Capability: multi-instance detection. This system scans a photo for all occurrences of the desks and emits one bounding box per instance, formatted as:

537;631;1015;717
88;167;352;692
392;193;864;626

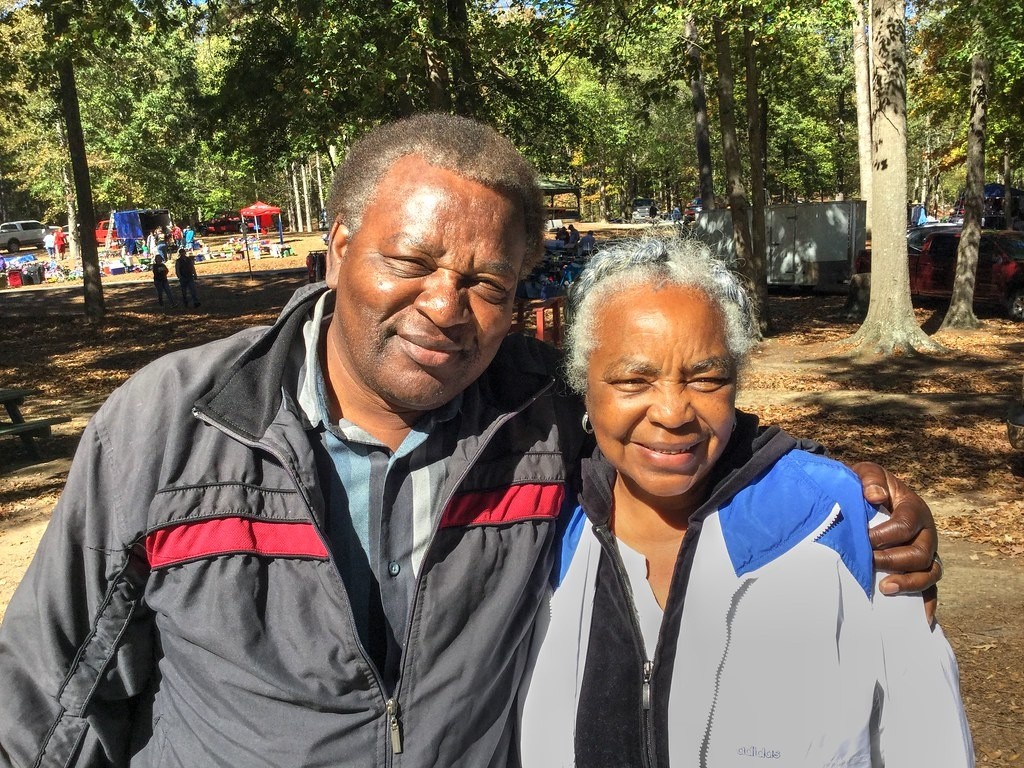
0;387;46;461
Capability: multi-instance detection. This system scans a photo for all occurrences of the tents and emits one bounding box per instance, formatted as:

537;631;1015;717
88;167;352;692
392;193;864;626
241;201;283;244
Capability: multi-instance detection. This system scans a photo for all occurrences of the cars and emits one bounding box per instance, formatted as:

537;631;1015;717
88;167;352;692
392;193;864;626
627;198;659;224
48;226;61;233
683;197;703;221
62;224;82;246
906;223;965;254
196;210;254;236
95;220;118;247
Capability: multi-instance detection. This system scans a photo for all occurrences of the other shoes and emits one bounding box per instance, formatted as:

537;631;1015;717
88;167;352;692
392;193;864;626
159;301;163;306
170;303;178;308
194;301;202;308
183;302;189;308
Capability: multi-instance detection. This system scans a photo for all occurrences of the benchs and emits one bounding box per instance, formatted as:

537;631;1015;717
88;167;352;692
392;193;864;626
0;416;72;447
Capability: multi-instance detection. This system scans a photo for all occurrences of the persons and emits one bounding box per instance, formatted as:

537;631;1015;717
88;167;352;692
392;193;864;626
625;203;634;223
153;255;177;308
72;224;81;258
671;204;680;227
175;249;201;307
513;235;975;768
54;227;68;260
649;202;659;217
0;112;941;768
142;223;194;263
555;225;595;255
42;229;56;259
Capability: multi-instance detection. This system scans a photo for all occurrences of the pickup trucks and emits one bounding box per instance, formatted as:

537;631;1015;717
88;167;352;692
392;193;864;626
856;228;1024;322
1;220;50;253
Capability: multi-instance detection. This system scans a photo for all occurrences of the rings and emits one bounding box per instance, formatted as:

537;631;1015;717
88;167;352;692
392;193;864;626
935;559;945;576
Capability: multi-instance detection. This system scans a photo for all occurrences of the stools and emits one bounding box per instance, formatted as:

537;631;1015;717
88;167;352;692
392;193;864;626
506;295;564;346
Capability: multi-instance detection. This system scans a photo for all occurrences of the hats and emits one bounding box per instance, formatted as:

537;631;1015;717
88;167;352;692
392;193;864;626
548;276;553;281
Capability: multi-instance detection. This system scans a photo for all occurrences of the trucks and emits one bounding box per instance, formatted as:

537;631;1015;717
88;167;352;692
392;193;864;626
681;199;867;286
